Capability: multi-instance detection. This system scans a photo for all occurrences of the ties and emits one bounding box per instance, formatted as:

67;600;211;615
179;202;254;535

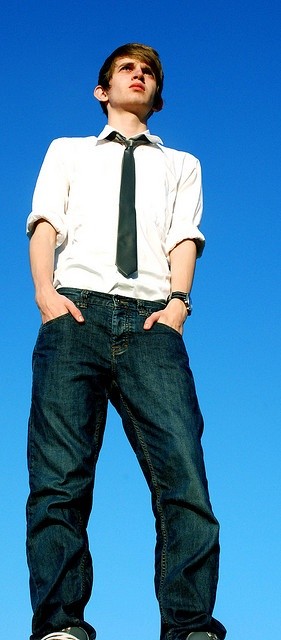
104;130;153;277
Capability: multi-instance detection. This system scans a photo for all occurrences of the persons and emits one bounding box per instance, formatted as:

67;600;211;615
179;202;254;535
25;43;228;640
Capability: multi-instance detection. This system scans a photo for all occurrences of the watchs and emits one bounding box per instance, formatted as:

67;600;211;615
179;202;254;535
167;291;192;316
